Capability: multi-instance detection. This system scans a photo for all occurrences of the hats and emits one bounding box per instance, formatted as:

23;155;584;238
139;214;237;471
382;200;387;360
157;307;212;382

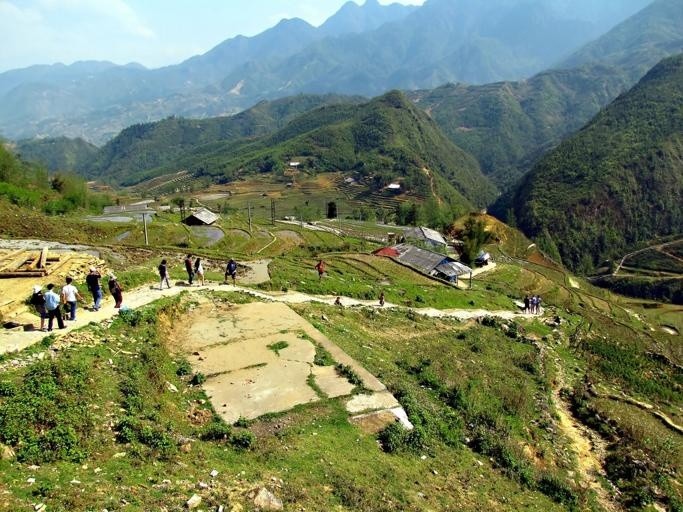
110;275;117;281
90;267;96;272
33;285;42;294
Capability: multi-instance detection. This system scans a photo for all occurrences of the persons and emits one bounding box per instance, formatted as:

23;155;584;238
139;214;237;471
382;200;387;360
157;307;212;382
225;258;237;287
31;285;46;331
524;295;541;314
185;254;195;284
158;260;171;290
195;258;204;287
109;275;122;308
61;277;83;320
43;284;67;331
315;259;325;280
86;267;101;311
379;293;384;306
334;297;342;305
